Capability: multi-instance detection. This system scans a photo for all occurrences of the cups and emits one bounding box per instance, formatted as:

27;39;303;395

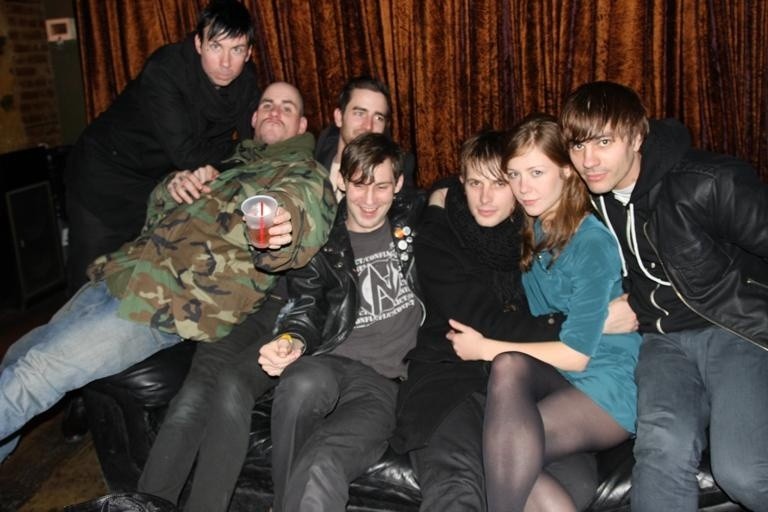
241;194;280;251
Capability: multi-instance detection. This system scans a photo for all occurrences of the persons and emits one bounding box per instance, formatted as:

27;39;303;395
386;130;640;512
138;77;392;511
562;81;768;512
259;133;448;511
0;81;336;460
63;1;264;443
445;110;642;511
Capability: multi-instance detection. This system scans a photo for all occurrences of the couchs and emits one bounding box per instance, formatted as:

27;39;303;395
80;308;753;512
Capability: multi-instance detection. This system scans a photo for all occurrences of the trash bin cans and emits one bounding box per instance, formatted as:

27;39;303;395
0;146;69;312
